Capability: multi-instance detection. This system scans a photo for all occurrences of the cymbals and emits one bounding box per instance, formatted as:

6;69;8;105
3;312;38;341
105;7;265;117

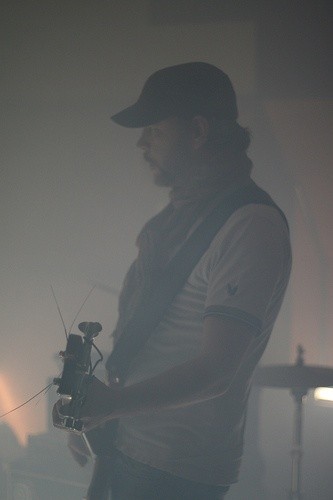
253;364;333;387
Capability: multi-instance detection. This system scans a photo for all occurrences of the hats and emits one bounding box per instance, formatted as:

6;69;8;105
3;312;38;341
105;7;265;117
109;61;239;128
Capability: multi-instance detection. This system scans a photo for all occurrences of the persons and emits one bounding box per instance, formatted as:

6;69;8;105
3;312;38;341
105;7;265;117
51;62;293;500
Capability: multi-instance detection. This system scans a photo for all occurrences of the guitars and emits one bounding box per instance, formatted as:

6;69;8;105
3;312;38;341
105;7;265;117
54;321;105;433
